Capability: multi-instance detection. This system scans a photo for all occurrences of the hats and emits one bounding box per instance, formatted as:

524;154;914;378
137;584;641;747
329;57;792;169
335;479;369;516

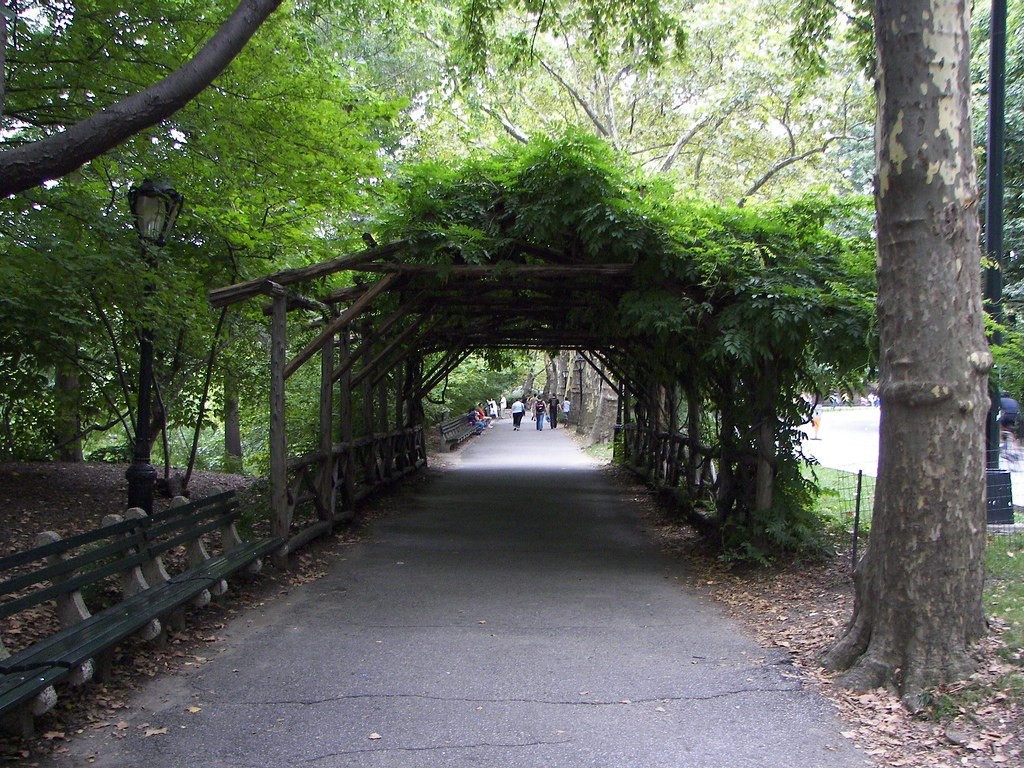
537;395;542;399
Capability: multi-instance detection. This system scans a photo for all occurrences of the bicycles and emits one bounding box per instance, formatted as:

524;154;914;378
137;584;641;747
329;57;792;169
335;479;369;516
1000;424;1023;472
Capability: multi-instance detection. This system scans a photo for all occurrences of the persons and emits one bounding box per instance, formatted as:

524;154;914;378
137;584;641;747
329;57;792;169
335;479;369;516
466;403;494;435
510;398;526;431
547;393;562;429
486;397;499;419
527;392;547;431
499;393;507;419
561;396;575;428
999;389;1021;447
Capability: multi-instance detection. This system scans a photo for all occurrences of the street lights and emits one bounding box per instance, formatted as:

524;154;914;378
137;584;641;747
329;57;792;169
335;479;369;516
117;173;186;534
561;366;568;389
575;356;587;430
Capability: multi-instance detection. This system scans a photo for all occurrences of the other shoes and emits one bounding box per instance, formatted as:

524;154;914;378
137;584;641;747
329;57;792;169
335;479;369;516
487;424;493;428
534;419;536;421
514;427;516;430
516;429;520;431
531;417;533;420
564;423;569;428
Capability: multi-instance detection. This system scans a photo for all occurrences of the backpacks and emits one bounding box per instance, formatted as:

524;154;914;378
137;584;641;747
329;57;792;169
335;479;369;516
550;398;557;407
536;400;546;412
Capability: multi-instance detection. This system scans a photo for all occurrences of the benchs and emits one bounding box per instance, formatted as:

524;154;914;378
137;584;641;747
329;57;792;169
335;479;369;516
439;413;489;453
0;489;280;739
